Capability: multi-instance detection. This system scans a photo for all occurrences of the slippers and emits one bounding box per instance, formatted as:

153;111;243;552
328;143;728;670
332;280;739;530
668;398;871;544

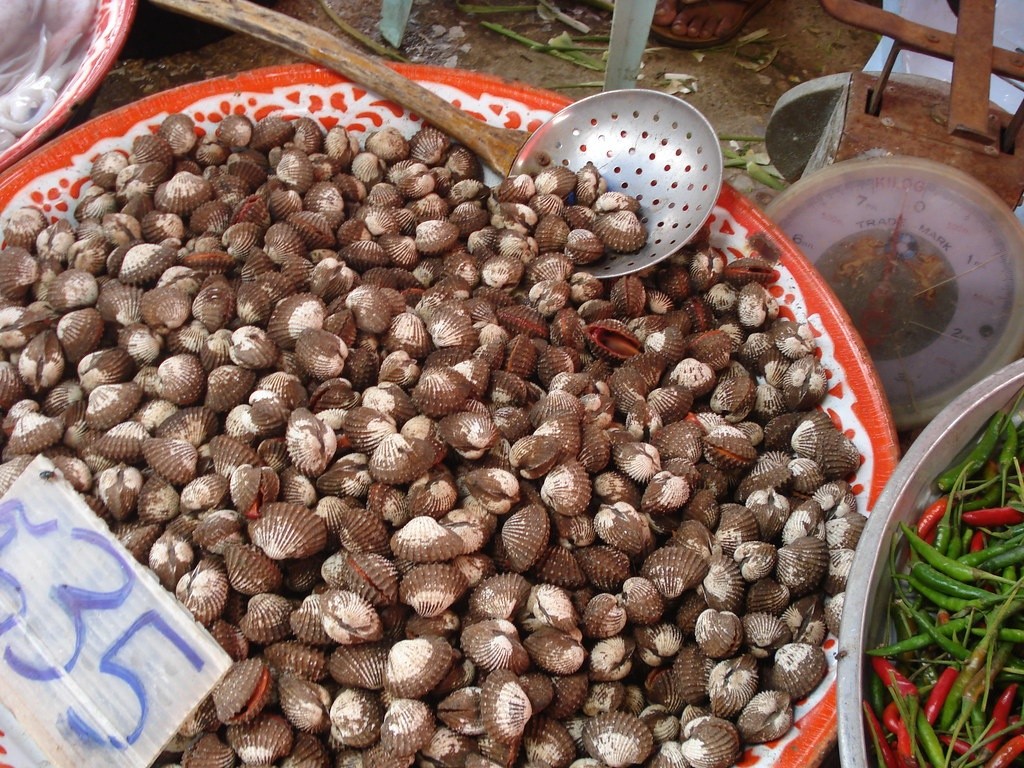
651;2;771;50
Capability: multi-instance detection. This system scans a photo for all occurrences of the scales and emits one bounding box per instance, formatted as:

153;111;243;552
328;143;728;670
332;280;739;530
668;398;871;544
759;0;1024;429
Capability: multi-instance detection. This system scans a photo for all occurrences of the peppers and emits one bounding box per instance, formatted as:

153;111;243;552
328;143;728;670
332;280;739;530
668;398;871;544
860;388;1024;768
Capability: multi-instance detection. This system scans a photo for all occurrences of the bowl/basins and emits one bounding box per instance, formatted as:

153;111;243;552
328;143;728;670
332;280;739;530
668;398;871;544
0;0;138;171
838;358;1024;768
0;63;900;768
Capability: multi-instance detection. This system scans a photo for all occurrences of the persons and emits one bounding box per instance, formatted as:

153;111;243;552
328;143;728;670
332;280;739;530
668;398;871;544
649;0;768;49
